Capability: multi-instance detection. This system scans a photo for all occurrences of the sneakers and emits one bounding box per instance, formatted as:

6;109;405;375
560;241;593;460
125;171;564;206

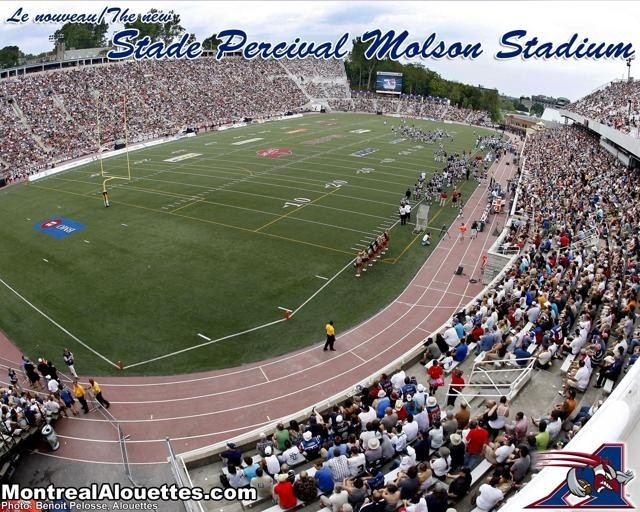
81;405;89;415
324;348;336;351
98;403;110;409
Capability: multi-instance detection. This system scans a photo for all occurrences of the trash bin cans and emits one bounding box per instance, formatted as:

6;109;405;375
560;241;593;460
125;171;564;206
41;424;60;451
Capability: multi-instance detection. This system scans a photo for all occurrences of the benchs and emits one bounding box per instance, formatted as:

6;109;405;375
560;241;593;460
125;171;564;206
1;400;61;456
223;295;630;511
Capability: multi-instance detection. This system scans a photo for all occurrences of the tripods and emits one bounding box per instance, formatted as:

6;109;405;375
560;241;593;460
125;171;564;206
439;227;450;240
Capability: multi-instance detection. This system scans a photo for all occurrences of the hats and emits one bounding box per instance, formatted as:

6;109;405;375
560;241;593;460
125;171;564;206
45;374;51;379
30;398;35;403
227;366;463;490
21;392;25;397
